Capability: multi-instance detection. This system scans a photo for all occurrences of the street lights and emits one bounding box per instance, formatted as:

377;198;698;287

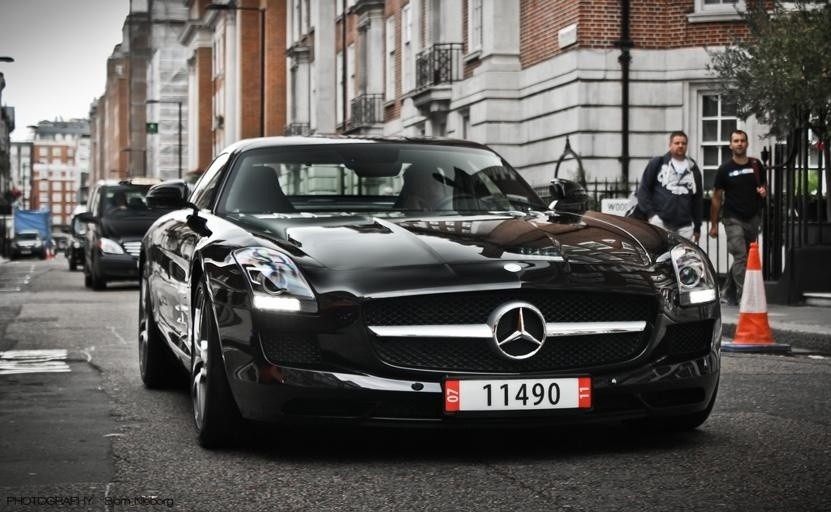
145;99;183;180
111;169;131;180
0;55;15;63
121;147;147;178
23;161;46;209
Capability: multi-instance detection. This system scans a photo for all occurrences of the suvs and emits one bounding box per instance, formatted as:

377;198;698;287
8;229;48;260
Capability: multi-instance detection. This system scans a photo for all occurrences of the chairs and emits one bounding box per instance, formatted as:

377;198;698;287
233;166;296;214
392;163;448;213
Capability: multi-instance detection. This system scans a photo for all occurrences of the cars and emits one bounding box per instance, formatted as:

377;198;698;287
138;133;724;447
80;177;190;292
48;223;73;251
61;206;87;272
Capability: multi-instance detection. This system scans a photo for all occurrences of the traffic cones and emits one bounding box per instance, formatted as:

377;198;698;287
51;248;56;260
720;242;793;354
45;248;52;261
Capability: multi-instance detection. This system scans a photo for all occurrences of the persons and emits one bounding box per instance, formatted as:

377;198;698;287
397;160;448;211
710;132;769;306
633;132;703;241
105;191;129;215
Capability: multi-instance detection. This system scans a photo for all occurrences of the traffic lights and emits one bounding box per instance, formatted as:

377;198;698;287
145;123;158;134
